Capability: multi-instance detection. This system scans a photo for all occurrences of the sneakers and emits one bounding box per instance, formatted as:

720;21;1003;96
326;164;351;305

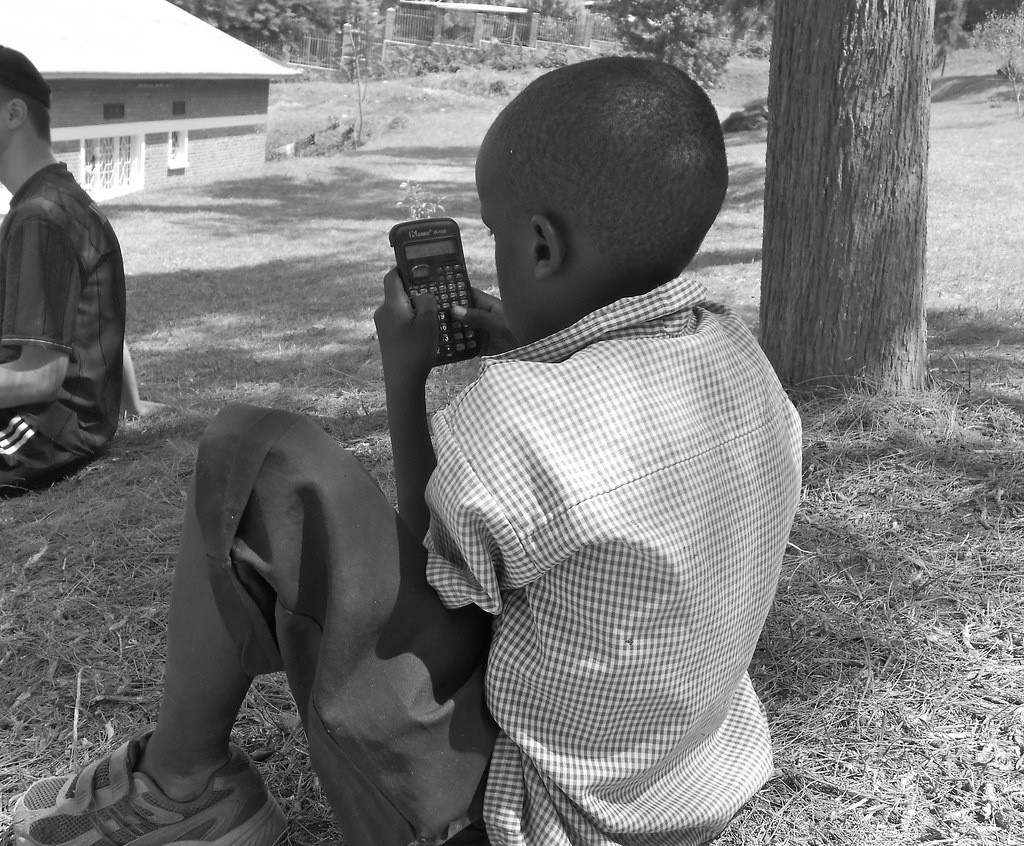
13;729;288;846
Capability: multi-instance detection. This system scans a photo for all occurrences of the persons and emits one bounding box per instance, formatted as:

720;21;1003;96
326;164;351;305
13;56;801;846
0;41;128;496
